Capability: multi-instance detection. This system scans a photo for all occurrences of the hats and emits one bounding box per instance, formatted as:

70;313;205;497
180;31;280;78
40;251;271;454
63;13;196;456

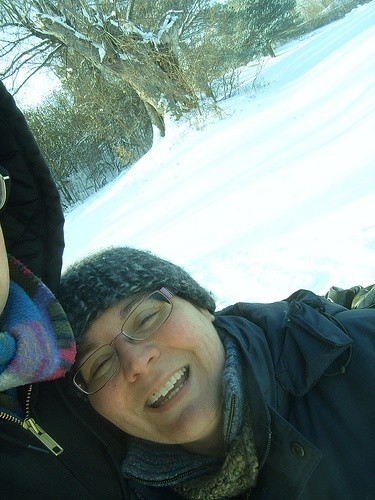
58;246;216;341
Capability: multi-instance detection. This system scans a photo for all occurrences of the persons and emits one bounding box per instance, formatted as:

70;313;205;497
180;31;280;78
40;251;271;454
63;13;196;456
0;82;375;500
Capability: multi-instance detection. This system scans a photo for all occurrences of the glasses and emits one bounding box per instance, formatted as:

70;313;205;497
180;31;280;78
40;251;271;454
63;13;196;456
0;174;9;209
72;287;174;395
